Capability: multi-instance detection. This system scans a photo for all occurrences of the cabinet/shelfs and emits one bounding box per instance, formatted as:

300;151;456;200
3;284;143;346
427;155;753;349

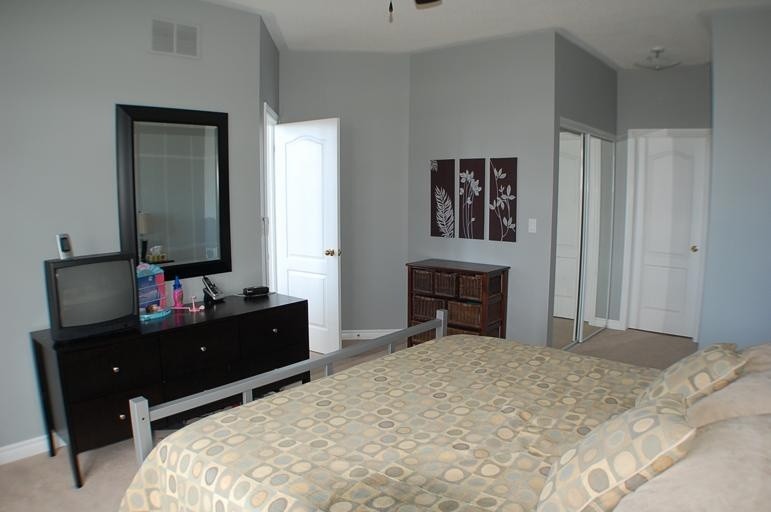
406;259;511;348
31;291;311;488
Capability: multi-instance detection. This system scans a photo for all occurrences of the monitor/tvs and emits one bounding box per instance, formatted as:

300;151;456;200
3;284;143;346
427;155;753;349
43;252;140;342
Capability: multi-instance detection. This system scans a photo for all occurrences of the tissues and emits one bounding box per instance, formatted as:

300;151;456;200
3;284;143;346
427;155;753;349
146;246;167;263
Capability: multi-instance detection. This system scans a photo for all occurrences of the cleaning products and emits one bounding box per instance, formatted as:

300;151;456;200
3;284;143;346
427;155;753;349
173;276;183;307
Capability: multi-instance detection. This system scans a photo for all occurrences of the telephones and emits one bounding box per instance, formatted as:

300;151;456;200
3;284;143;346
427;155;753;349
202;272;225;304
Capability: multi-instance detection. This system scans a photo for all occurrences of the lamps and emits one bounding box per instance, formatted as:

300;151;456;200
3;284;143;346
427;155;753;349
136;213;153;259
635;46;680;70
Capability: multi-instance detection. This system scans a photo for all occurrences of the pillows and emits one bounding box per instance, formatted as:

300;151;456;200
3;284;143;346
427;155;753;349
537;343;771;512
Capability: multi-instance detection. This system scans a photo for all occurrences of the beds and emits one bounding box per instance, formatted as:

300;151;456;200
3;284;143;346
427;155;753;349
118;308;771;512
